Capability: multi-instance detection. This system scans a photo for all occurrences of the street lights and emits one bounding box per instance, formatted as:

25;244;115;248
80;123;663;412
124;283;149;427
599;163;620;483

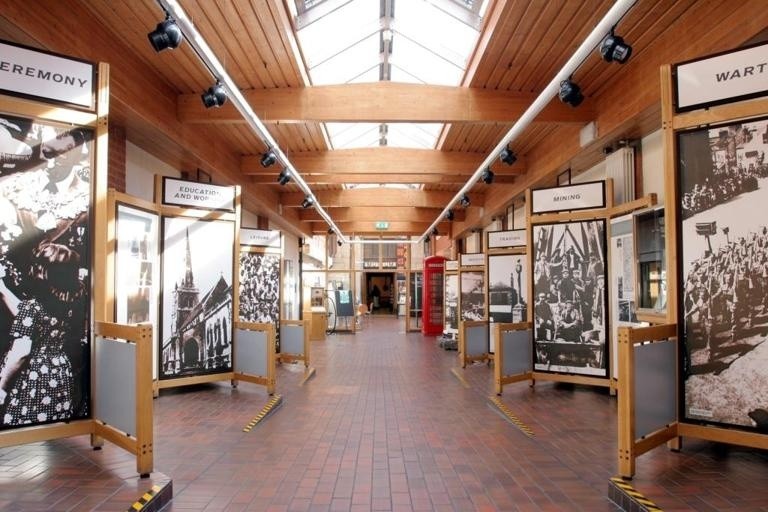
515;260;523;304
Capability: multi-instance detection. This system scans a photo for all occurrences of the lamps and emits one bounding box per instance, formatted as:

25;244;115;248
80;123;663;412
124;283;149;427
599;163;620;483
481;166;497;184
259;144;313;211
599;33;633;64
201;76;228;108
556;80;584;108
460;192;470;207
147;12;181;52
501;145;516;166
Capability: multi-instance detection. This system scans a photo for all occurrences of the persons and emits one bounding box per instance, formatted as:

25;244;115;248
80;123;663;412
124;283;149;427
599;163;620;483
0;118;90;430
239;254;280;322
680;150;768;372
534;245;603;342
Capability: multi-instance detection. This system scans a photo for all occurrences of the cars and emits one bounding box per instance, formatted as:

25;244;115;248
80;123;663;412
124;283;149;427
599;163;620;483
490;286;512;321
